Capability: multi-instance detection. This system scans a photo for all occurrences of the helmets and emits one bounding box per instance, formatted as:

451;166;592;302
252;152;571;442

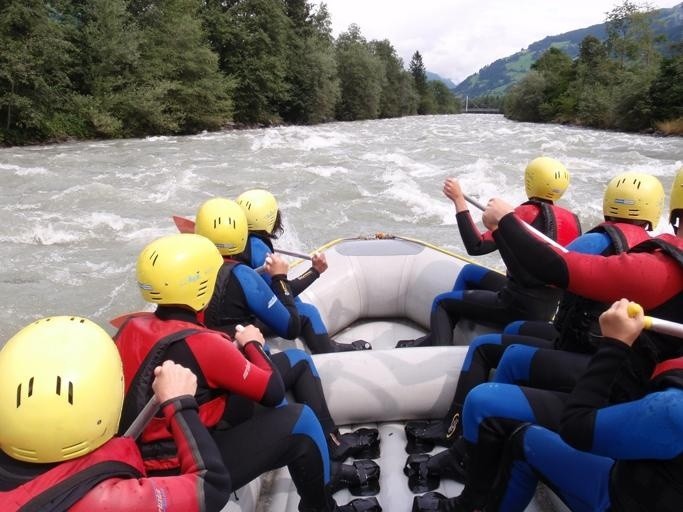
524;157;571;202
237;188;278;235
669;167;683;234
0;317;124;462
195;198;248;256
138;234;224;311
604;173;665;231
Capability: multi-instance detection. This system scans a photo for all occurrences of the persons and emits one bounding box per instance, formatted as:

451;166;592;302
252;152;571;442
396;156;581;347
114;233;379;512
1;315;231;512
195;197;378;462
410;300;683;511
233;190;372;353
400;165;683;493
405;171;665;454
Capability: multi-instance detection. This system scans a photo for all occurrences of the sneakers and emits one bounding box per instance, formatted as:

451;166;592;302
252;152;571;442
328;340;371;352
412;492;478;511
328;428;378;463
333;498;382;512
330;460;380;497
396;335;433;347
405;415;463;447
410;450;477;483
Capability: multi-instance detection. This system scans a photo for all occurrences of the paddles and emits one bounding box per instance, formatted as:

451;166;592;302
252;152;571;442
172;215;314;262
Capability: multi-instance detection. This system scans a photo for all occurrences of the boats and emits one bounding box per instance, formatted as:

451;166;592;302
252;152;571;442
210;233;562;511
